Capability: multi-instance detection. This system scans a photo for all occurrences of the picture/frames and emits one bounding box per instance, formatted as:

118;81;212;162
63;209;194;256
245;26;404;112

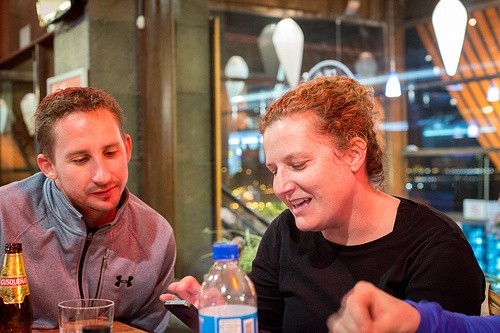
46;67;88;96
212;2;392;275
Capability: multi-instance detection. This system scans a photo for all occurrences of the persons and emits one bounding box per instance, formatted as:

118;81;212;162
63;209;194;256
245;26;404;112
0;88;177;333
159;76;486;332
326;280;500;332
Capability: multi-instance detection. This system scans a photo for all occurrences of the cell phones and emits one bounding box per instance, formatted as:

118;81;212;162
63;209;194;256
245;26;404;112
164;300;199;331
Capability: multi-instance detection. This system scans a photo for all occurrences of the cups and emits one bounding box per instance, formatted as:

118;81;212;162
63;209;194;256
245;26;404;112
58;299;114;333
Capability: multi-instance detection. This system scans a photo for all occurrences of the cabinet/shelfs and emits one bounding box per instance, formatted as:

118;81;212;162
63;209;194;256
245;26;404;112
0;0;53;187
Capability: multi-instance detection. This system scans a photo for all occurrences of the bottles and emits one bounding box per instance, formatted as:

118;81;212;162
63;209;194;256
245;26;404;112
197;244;259;333
0;241;34;333
